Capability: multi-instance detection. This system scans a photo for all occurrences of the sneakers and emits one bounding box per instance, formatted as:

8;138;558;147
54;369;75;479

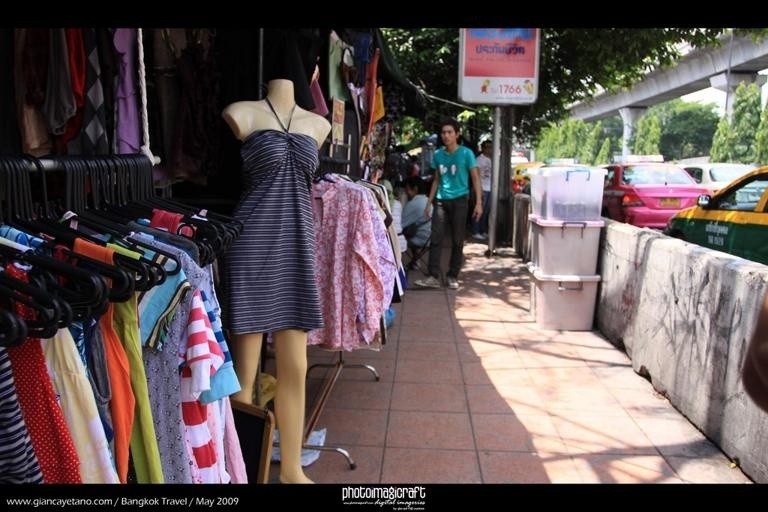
412;276;441;288
445;274;459;289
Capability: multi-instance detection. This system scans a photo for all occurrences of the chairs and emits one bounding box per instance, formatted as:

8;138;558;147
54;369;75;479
407;218;434;275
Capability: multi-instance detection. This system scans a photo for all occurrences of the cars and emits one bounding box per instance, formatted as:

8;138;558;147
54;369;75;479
597;161;767;266
511;151;577;194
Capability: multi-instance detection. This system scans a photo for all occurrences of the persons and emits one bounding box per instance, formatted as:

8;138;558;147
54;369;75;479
409;116;484;291
373;134;494;272
220;76;336;485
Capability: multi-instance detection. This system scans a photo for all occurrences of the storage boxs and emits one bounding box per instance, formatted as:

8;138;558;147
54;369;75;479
526;165;608;331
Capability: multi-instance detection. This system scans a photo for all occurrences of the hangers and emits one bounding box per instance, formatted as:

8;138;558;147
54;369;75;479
0;150;244;350
312;155;352;188
